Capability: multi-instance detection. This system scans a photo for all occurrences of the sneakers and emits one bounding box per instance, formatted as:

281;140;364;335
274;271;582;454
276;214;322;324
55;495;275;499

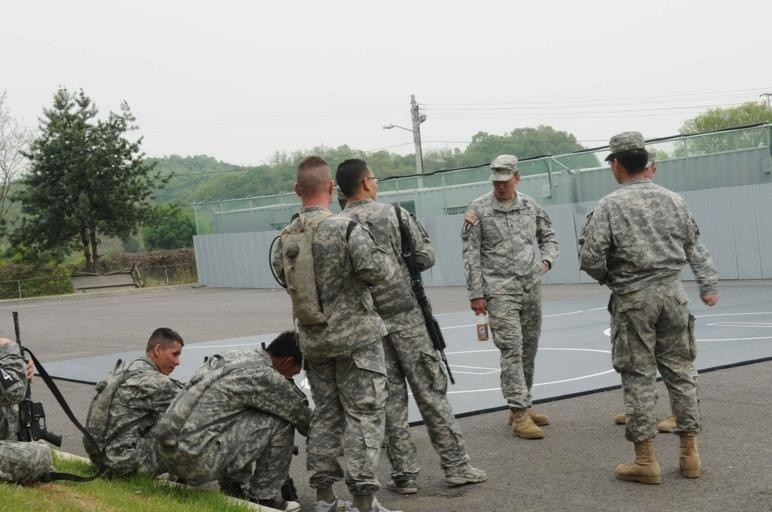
343;496;403;512
443;461;488;484
312;496;350;511
250;496;301;511
383;476;417;493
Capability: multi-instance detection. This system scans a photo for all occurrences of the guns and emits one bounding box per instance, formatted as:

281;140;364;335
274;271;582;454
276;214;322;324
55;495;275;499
260;340;298;498
403;249;455;386
12;311;62;448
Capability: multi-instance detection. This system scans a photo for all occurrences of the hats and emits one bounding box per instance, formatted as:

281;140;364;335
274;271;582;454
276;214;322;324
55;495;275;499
644;150;657;169
604;131;644;161
488;154;518;182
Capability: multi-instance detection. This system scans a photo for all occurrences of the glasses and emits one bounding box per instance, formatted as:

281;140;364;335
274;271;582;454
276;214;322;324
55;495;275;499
362;176;379;184
607;160;613;166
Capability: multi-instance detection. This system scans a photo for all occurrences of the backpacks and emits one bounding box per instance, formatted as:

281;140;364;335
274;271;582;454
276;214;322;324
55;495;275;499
155;353;267;448
281;212;352;346
368;203;415;319
83;357;160;455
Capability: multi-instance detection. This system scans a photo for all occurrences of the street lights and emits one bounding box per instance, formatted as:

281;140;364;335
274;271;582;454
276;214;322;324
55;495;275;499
382;94;428;189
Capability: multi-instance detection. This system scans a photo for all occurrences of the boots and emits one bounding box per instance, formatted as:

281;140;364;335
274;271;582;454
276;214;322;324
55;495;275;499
657;416;677;432
510;410;544;439
507;408;549;425
615;439;662;483
679;431;701;478
614;412;659;425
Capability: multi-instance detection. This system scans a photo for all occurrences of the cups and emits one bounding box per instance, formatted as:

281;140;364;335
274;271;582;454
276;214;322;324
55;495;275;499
476;312;488;341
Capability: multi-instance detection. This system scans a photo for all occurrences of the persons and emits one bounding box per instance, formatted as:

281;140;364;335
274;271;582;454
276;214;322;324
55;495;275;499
614;146;719;432
156;332;314;512
272;156;393;512
577;131;702;484
336;160;488;495
337;185;388;450
82;328;187;478
460;155;559;438
0;337;50;484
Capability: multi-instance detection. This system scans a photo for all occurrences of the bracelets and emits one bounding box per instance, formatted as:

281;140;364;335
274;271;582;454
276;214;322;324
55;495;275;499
544;260;550;266
